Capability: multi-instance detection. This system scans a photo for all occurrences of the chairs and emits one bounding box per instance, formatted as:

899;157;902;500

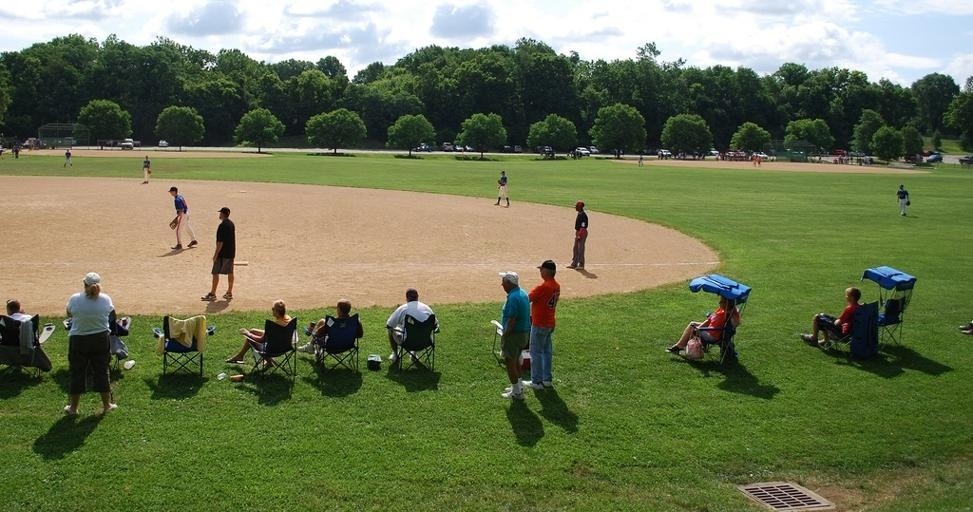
860;266;916;355
63;315;124;374
150;312;212;377
316;314;365;372
0;312;44;381
817;299;882;352
679;271;750;369
398;313;438;369
242;315;300;385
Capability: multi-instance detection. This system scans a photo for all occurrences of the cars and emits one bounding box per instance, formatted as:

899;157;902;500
847;149;865;157
442;142;454;151
906;154;923;163
834;145;846;157
657;150;671;156
158;140;167;147
708;148;718;155
413;143;432;152
464;145;475;152
133;140;141;147
727;150;767;157
923;150;938;156
926;155;943;163
454;144;464;151
22;137;77;148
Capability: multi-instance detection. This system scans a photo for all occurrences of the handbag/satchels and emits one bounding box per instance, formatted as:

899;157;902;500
685;327;703;359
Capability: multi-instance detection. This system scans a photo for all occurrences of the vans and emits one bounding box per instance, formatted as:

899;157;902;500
614;147;624;154
543;144;551;152
534;144;544;152
587;146;598;154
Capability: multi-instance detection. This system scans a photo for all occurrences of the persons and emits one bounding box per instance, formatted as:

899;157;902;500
638;155;643;165
896;185;908;215
958;320;972;333
63;150;72;168
527;260;560;389
63;272;118;414
494;171;509;208
799;288;861;346
614;150;624;159
0;144;2;158
297;299;350;354
167;187;197;250
26;137;40;149
200;207;234;301
0;300;37;350
657;151;706;161
498;271;530;400
716;152;750;161
386;289;439;361
224;300;292;363
666;295;739;354
14;144;18;158
833;151;874;165
142;156;150;183
752;153;761;165
565;202;588;270
572;150;583;160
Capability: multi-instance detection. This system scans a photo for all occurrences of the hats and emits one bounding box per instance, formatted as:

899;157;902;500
82;272;100;286
498;271;517;285
167;186;177;192
536;260;555;270
216;207;230;215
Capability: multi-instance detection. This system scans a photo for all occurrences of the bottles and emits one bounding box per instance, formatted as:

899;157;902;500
307;321;315;332
229;374;244;382
121;319;126;325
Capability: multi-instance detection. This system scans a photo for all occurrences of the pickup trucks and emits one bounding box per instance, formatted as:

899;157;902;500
785;149;805;157
958;156;972;165
121;139;133;150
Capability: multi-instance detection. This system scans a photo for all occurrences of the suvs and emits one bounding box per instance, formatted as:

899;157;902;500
511;145;521;153
575;147;590;157
502;143;511;153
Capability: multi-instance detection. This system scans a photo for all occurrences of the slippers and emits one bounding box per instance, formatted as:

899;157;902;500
224;358;244;364
667;345;683;351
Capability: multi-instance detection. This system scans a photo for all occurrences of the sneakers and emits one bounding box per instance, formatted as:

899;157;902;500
501;381;551;399
223;293;232;299
188;241;197;247
65;405;78;415
200;293;216;300
103;404;117;413
171;245;181;249
799;333;817;345
297;344;314;354
817;339;831;347
389;354;396;361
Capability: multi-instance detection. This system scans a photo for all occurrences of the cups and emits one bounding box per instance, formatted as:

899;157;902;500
123;359;135;369
216;372;225;380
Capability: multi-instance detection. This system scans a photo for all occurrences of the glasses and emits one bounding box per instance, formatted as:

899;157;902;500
272;307;275;310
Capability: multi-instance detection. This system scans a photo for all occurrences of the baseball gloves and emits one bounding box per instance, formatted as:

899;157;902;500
148;169;152;174
906;200;910;206
171;217;179;230
